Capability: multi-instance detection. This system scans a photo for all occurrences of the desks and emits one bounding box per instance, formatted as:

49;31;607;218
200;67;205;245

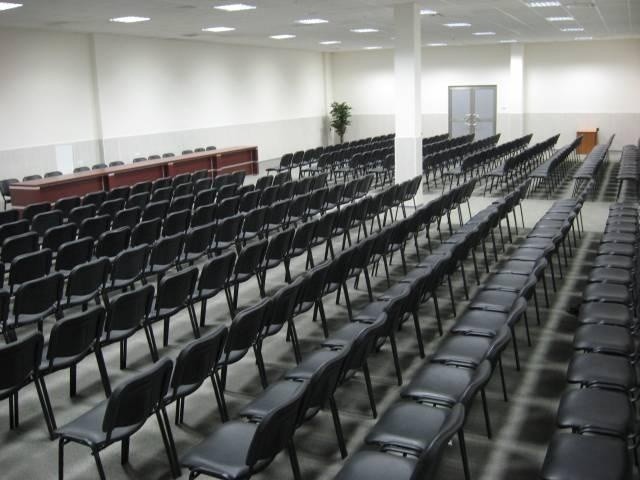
9;142;262;211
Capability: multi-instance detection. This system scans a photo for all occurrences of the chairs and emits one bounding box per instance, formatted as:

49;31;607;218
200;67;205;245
422;130;639;201
152;323;229;470
333;403;467;479
566;196;640;386
265;132;395;189
402;325;513;402
553;385;640;433
2;168;380;341
317;313;401;420
216;297;272;391
1;331;55;442
35;303;110;430
54;357;179;479
100;283;159;372
536;434;640;480
2;142;217;211
363;356;492;479
179;377;311;478
148;177;599;368
236;345;353;478
